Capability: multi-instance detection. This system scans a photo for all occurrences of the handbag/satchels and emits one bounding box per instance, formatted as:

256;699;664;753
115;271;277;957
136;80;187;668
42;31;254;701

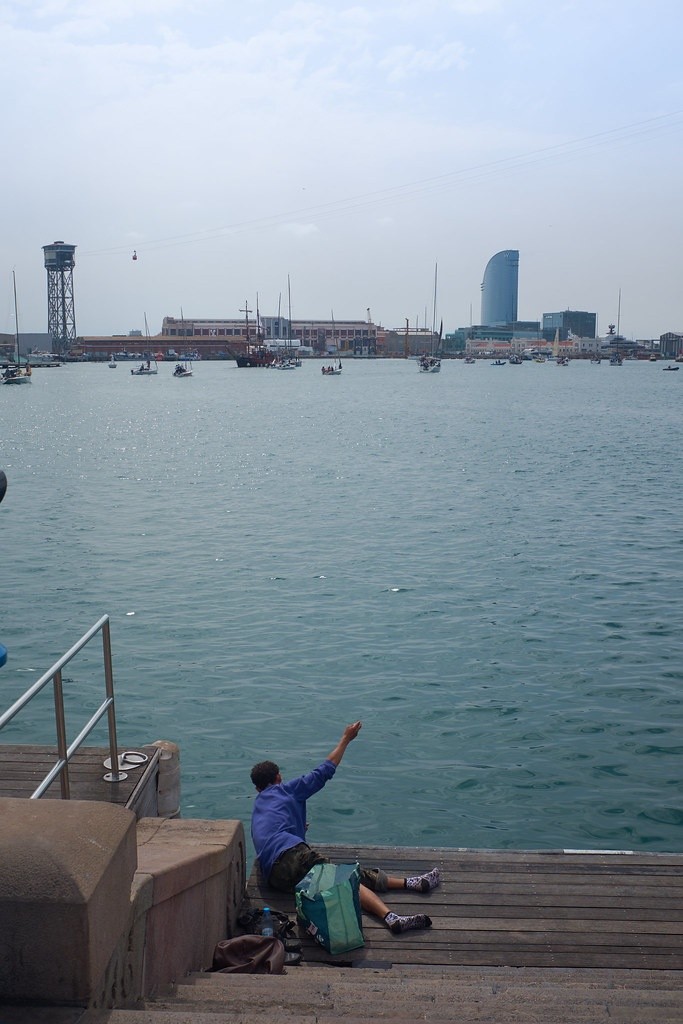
208;935;285;976
294;862;366;957
235;907;295;939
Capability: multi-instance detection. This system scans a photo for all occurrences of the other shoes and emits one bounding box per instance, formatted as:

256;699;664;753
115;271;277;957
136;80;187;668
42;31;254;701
283;938;302;950
284;952;302;964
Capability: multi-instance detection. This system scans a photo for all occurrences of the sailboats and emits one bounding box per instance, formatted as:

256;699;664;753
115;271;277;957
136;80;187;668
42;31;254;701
509;295;523;364
417;263;442;374
609;288;624;366
534;321;545;363
131;312;158;375
322;308;343;376
173;306;194;378
225;274;303;370
464;303;476;364
0;270;31;385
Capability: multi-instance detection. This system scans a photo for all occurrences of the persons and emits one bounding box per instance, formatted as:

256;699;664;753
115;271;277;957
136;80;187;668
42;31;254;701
140;363;145;371
24;362;32;376
251;721;440;932
422;358;432;366
322;365;333;373
2;369;18;378
173;362;185;376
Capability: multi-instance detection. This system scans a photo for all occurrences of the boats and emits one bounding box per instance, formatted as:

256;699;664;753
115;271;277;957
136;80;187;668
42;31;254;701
591;354;601;365
492;361;506;365
518;349;553;361
626;354;638;360
663;366;680;371
676;354;683;362
557;357;569;365
650;354;656;361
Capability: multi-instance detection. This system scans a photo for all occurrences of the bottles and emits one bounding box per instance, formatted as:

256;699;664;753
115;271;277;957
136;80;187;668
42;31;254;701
261;907;273;937
